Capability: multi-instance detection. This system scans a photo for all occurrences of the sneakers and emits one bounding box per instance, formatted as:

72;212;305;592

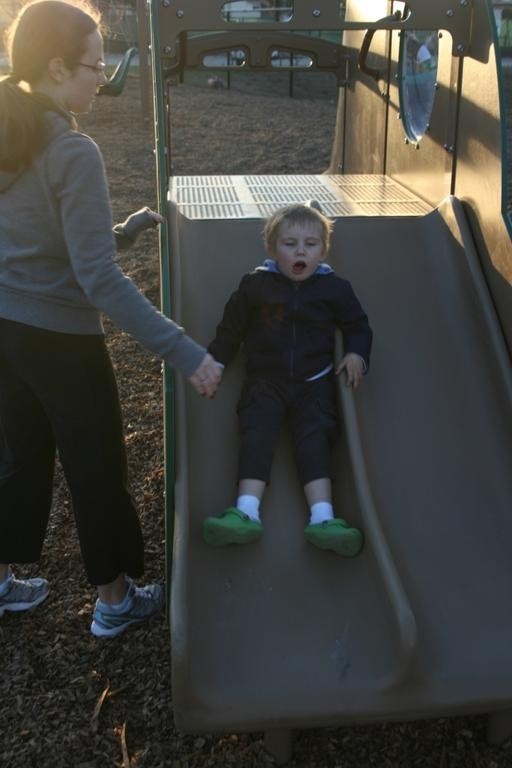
1;568;53;618
87;577;167;638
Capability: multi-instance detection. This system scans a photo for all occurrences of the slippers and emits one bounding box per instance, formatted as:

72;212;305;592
301;516;365;560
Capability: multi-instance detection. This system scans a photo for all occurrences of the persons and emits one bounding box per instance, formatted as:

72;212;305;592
189;201;373;563
0;0;221;644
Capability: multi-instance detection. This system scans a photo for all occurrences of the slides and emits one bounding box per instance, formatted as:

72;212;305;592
98;46;139;97
165;200;512;732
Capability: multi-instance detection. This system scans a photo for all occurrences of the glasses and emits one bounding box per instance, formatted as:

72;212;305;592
68;57;108;77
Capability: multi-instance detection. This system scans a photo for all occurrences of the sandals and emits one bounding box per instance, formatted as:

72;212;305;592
200;504;267;551
